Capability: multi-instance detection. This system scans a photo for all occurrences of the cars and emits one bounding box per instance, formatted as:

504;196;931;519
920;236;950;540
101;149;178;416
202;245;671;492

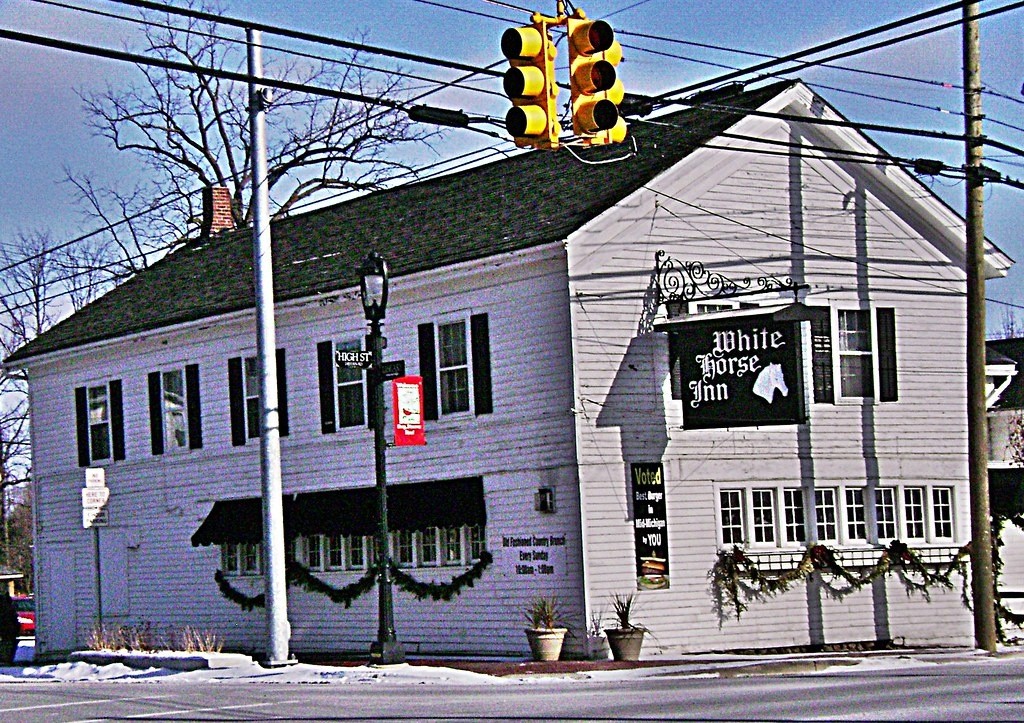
10;593;36;638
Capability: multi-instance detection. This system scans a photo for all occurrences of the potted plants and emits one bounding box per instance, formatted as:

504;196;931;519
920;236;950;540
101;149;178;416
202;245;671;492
515;592;586;661
601;591;652;661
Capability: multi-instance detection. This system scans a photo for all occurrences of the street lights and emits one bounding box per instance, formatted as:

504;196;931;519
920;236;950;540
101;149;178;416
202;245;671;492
353;252;406;669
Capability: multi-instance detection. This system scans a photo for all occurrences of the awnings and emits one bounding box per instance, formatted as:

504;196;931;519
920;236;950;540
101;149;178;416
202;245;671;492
191;476;487;547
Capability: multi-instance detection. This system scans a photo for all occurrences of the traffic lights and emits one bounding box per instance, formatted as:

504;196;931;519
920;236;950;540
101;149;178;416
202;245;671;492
499;12;561;153
567;17;618;148
603;39;630;147
